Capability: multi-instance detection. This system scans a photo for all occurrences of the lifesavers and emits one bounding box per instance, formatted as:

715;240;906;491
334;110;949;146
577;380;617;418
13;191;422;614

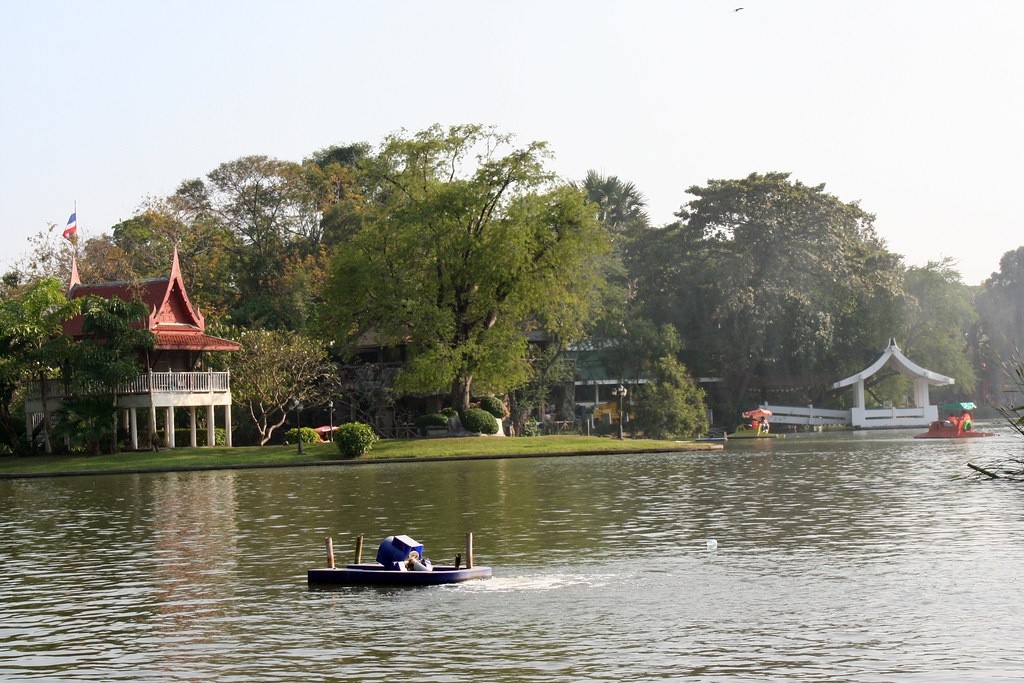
963;420;971;432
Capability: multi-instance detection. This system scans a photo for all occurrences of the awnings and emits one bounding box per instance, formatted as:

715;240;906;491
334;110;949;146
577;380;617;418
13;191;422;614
746;409;772;418
942;402;977;411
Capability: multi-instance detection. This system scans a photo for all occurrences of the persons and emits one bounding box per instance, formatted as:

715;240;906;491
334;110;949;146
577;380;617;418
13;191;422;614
743;415;769;433
405;551;433;572
948;410;970;427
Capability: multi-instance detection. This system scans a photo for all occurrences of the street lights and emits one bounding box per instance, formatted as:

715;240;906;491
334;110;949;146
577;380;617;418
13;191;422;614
295;406;304;454
329;401;334;442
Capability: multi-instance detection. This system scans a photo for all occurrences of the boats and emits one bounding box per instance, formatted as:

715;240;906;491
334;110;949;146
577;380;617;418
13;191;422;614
305;534;492;588
912;402;995;439
725;408;786;438
